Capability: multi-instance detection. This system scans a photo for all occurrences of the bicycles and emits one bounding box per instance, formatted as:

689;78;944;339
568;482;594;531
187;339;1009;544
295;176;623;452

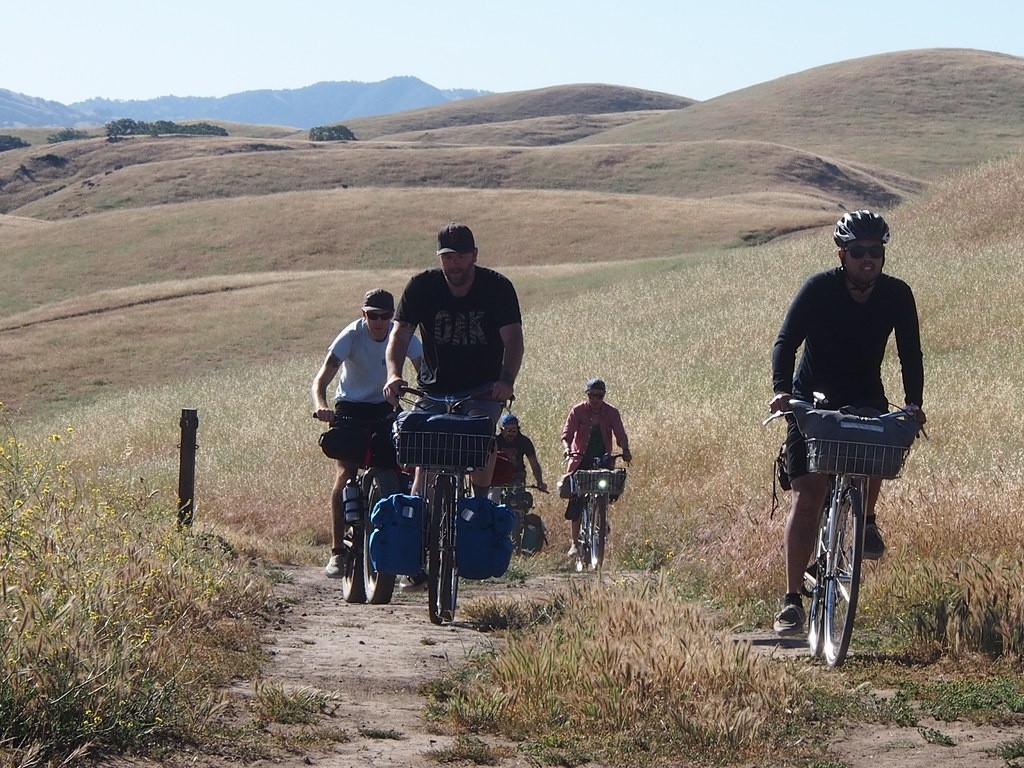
563;452;634;572
487;483;550;556
761;391;930;667
390;386;515;624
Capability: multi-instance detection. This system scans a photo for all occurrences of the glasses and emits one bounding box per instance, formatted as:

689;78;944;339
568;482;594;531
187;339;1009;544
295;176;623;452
846;245;885;258
588;393;603;399
366;312;390;320
502;427;517;432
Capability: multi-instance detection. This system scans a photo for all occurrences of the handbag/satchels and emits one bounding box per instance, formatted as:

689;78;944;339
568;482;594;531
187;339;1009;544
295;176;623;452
770;441;792;520
557;473;570;498
319;427;387;464
370;493;425;576
789;399;921;475
502;492;533;508
456;497;515;580
521;513;548;558
390;410;493;468
492;457;513;482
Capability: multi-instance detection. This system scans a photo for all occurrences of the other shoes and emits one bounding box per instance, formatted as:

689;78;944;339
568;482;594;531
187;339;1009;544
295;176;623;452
325;555;344;577
568;544;578;557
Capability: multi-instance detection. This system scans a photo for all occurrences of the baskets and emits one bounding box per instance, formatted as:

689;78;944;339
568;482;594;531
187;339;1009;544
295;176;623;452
395;431;496;472
574;472;626;495
805;438;911;479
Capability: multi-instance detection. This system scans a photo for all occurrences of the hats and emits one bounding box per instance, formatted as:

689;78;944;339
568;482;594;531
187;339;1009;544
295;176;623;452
437;222;475;255
362;289;393;312
502;415;516;426
584;379;605;391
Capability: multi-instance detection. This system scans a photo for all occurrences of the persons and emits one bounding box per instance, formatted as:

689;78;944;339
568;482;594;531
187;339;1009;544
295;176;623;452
770;209;924;636
382;221;525;500
491;414;547;502
559;378;632;558
310;287;425;580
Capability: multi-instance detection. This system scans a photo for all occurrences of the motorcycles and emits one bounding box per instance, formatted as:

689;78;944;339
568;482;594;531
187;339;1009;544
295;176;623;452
312;410;404;604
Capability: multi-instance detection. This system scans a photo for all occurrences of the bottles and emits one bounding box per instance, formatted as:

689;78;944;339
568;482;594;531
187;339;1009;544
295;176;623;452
343;479;361;523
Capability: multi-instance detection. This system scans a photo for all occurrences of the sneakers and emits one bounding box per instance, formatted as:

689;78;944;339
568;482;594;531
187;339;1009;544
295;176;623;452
864;524;885;554
773;603;806;634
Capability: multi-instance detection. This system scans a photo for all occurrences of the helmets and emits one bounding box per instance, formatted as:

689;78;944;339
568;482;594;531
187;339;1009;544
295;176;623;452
833;209;890;247
